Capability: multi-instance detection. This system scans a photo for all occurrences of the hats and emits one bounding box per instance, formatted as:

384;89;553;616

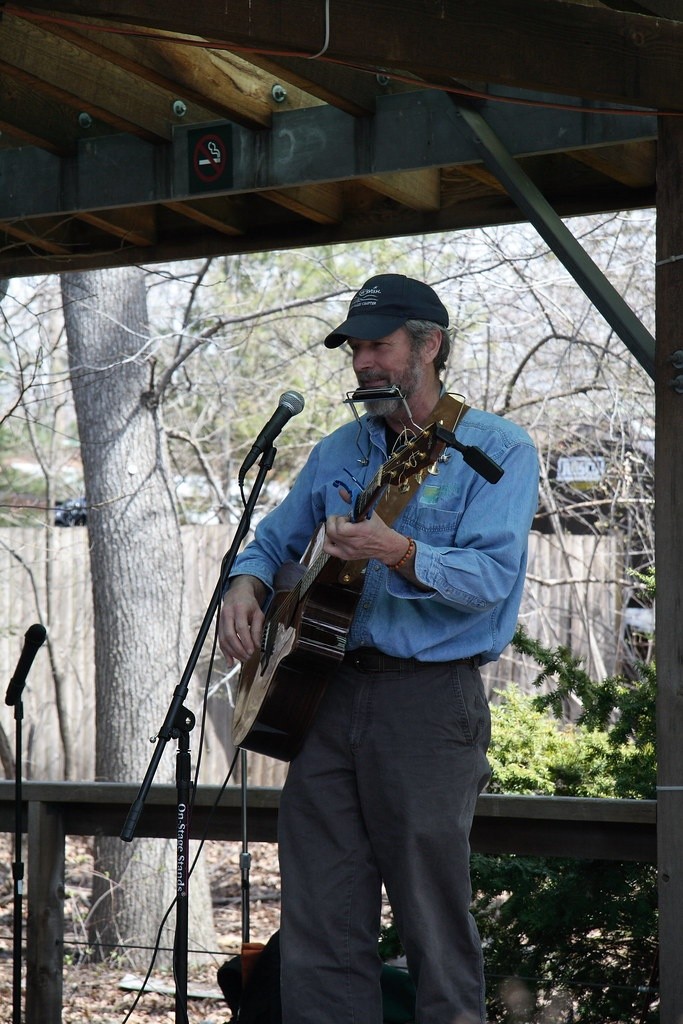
324;274;449;349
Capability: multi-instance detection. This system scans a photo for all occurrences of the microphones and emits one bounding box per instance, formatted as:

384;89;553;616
239;390;305;486
5;624;47;706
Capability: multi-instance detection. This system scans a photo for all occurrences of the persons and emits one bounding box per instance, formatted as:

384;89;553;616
220;273;539;1024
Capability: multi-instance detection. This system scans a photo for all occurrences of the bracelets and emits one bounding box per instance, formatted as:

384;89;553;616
387;537;413;570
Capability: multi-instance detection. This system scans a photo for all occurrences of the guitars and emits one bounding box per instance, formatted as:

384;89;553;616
232;418;444;763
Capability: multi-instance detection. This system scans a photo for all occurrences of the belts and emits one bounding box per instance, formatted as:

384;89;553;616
341;647;479;671
